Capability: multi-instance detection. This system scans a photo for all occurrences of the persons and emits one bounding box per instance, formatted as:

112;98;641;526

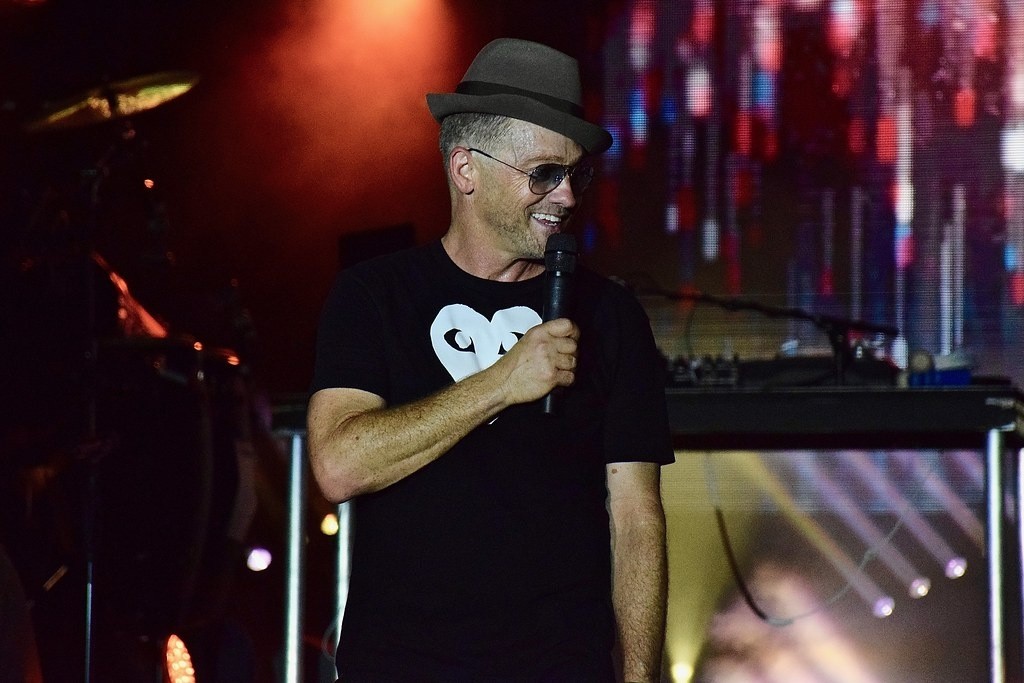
307;39;675;683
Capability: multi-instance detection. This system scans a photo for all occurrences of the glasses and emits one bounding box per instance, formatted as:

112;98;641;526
468;147;594;197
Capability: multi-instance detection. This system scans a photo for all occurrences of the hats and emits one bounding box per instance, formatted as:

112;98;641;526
426;37;614;156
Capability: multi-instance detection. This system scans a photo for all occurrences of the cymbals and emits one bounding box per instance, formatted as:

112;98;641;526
21;66;200;137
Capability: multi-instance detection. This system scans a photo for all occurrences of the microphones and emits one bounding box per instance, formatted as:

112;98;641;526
543;232;582;413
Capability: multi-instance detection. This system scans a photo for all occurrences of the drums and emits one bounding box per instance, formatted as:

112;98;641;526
70;333;264;635
